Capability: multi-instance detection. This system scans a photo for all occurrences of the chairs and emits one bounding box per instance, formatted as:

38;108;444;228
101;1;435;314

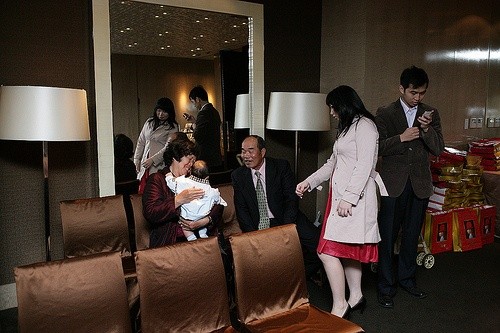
225;223;366;333
13;250;132;333
211;183;245;299
128;194;150;251
58;195;138;287
132;236;240;333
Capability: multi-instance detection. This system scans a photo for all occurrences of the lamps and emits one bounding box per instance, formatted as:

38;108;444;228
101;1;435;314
233;93;250;129
266;90;331;240
0;85;91;261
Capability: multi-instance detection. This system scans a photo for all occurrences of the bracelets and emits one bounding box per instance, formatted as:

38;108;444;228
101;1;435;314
207;216;211;223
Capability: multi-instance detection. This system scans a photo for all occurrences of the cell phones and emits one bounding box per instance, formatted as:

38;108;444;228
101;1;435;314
182;113;190;120
422;110;434;119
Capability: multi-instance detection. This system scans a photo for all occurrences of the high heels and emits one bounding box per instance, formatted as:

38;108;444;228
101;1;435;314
351;296;367;314
342;303;351;320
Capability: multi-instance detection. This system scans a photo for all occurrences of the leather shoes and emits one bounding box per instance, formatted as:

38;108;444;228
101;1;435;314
376;295;394;307
406;287;427;298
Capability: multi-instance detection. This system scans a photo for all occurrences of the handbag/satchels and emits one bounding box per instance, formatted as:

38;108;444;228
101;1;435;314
421;137;500;255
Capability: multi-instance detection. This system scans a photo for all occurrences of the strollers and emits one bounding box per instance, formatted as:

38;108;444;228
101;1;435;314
368;228;436;273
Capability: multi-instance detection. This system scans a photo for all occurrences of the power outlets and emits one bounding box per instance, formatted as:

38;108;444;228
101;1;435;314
464;119;469;130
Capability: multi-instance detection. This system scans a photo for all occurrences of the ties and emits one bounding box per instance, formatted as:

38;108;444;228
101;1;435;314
407;110;414;128
254;172;270;230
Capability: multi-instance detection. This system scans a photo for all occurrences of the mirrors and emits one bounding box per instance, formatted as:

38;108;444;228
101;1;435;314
92;0;265;250
483;0;500;141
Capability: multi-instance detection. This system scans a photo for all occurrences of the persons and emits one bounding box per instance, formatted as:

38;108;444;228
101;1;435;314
164;132;190;151
114;134;137;183
372;64;444;309
231;136;320;286
295;85;381;320
165;160;228;242
182;87;221;185
133;97;179;194
142;140;228;256
